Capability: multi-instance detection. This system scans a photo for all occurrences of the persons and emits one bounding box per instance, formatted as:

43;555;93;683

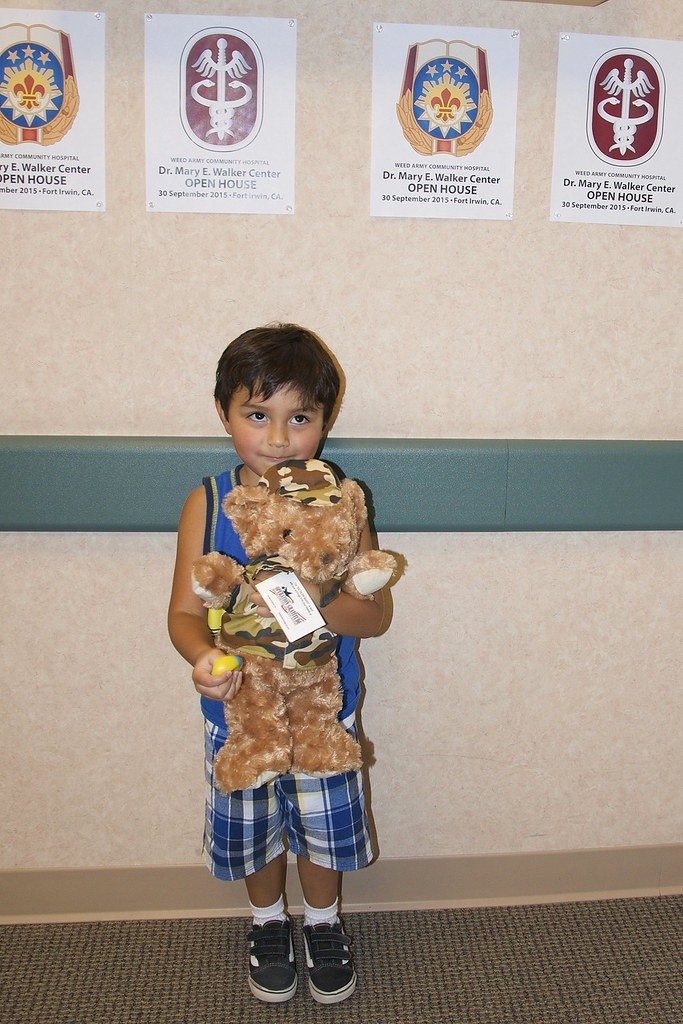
167;320;394;1006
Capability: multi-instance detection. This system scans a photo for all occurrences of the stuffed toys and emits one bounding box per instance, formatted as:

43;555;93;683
189;458;399;793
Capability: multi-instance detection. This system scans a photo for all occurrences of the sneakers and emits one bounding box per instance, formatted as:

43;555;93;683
302;916;356;1003
246;909;297;1002
